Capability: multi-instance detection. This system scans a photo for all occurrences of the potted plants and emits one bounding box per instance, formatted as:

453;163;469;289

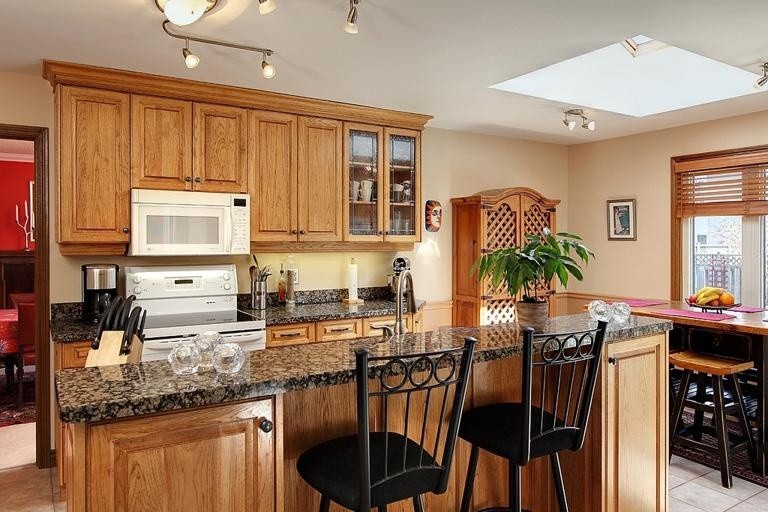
466;225;594;334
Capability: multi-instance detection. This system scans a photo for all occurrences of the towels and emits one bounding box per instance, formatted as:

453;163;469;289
624;297;667;307
653;304;736;329
726;300;766;315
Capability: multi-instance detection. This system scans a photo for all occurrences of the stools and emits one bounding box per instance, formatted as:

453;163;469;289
667;347;753;489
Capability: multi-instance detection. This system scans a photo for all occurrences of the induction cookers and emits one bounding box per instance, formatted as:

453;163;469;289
130;300;266;336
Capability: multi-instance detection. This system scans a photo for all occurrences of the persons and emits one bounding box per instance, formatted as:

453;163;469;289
614;206;623;234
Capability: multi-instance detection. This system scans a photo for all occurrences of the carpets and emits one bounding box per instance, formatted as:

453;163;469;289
0;368;43;429
658;362;767;492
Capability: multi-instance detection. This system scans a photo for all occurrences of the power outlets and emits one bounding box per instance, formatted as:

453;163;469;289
288;267;299;285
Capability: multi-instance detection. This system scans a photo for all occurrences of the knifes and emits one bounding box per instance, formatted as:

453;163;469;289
90;295;149;354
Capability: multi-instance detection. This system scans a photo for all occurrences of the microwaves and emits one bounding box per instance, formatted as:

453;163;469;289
130;187;253;257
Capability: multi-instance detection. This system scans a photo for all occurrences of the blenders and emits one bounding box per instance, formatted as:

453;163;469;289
387;258;411;302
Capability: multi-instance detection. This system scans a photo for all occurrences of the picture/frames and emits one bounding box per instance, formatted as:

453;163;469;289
606;198;637;241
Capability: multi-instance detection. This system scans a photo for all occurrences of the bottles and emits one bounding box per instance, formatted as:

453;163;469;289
287;254;296;304
348;257;358;299
277;270;287;305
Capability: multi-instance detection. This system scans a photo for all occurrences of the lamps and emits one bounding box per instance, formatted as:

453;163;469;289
560;108;595;133
751;60;767;91
152;0;365;82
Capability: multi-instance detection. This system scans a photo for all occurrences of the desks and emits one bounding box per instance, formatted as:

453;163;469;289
0;309;20;355
582;297;767;481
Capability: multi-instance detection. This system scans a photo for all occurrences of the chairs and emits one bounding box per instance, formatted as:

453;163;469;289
7;293;35;308
5;304;38;416
455;317;610;512
291;333;482;512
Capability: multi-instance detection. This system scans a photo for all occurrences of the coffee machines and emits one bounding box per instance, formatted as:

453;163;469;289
80;265;118;325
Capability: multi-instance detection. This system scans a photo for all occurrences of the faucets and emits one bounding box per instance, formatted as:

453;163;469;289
372;335;405;356
371;270;419;335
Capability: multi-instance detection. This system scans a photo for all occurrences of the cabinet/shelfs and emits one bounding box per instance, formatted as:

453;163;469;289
266;324;312;348
446;184;564;329
39;59;133;258
243;89;341;253
0;160;36;255
51;341;91;502
339;97;434;256
599;332;668;512
130;71;247;197
314;319;360;339
361;309;423;338
67;386;286;512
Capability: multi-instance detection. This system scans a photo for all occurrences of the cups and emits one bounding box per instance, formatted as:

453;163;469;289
587;300;632;323
350;179;411;204
369;208;413;235
254;281;266;310
169;332;242;376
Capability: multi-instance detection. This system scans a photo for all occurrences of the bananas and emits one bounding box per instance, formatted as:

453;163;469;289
696;287;722;305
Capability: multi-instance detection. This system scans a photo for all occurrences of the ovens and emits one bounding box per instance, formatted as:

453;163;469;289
140;329;266;362
